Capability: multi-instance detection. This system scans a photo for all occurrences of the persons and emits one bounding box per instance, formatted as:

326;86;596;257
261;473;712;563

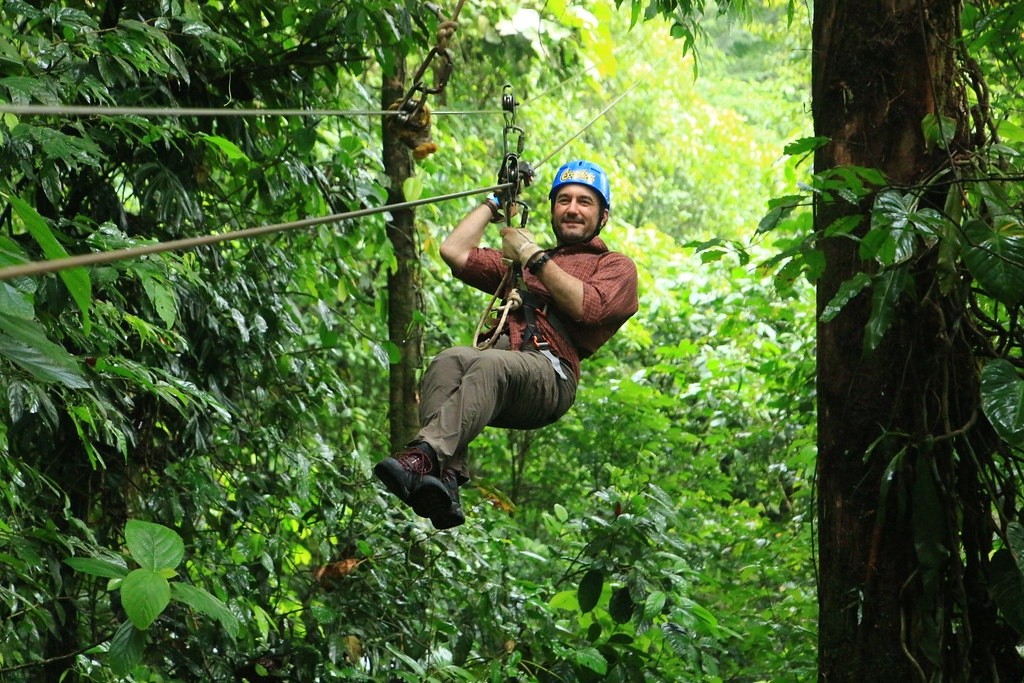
374;160;640;526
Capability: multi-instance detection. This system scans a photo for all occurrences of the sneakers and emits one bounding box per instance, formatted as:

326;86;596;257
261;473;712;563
373;441;465;531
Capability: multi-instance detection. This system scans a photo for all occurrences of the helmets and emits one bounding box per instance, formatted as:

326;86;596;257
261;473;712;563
548;160;610;210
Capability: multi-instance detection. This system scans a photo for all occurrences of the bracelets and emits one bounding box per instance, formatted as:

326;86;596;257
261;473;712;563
528;252;550;276
484;199;501;218
